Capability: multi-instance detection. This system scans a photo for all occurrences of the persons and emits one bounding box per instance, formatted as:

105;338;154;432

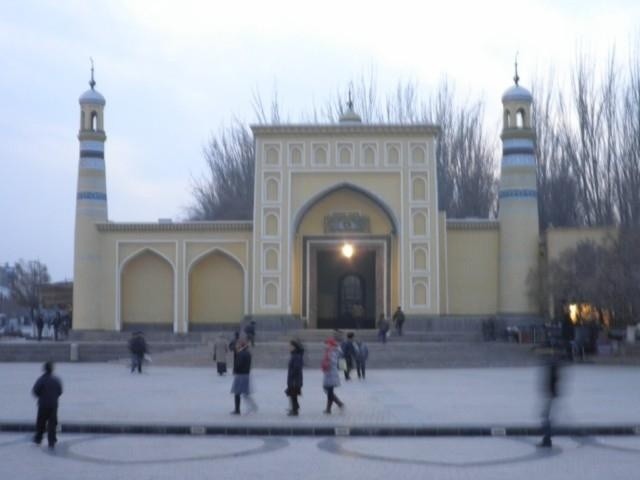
131;330;147;373
526;339;597;448
32;309;73;343
334;328;368;381
392;305;405;336
228;332;241;373
320;337;346;414
229;338;258;416
31;361;64;451
213;332;230;376
129;331;138;368
286;338;304;416
243;320;256;348
478;305;604;367
377;312;390;342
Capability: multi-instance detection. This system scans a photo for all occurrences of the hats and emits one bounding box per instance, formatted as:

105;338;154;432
236;340;246;347
326;337;337;345
291;338;302;349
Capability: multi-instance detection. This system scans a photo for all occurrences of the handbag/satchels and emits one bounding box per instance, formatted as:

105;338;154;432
339;358;347;371
